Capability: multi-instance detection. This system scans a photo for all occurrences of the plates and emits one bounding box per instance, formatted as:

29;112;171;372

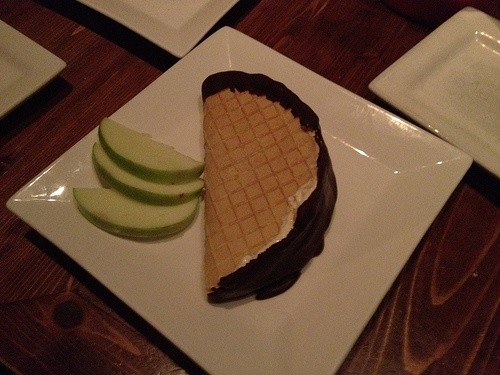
368;7;500;178
0;19;66;117
6;26;472;375
78;1;241;59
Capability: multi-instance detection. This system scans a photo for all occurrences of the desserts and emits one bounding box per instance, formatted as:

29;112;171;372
200;70;339;305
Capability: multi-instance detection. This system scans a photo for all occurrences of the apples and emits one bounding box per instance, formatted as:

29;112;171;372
70;118;206;240
